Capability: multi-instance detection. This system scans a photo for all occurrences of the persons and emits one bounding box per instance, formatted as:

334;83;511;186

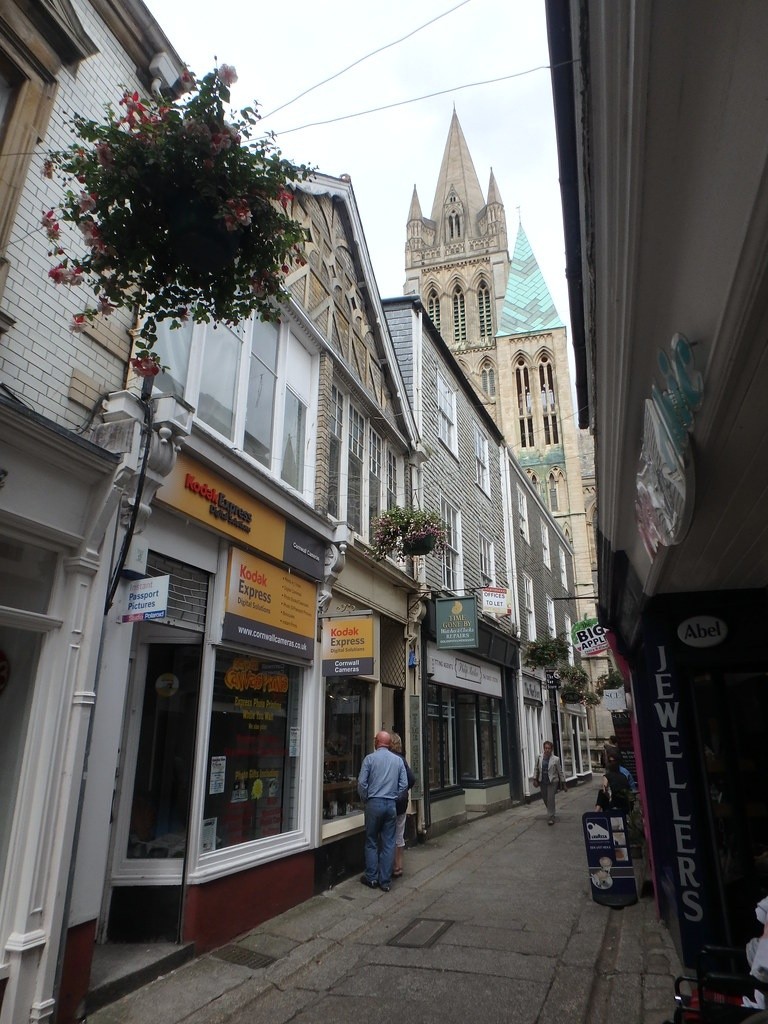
533;741;568;825
358;731;408;892
596;736;636;814
389;733;416;876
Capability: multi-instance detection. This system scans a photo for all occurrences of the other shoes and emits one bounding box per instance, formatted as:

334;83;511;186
393;868;404;878
381;886;390;892
361;876;377;888
548;819;554;824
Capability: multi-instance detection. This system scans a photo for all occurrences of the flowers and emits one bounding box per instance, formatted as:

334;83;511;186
38;56;319;376
364;505;451;562
523;631;624;707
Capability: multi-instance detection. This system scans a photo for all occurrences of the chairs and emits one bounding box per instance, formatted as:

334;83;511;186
664;945;768;1024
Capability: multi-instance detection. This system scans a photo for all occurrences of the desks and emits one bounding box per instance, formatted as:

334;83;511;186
321;779;357;803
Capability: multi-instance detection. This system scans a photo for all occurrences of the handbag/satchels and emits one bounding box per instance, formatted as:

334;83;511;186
534;757;540;788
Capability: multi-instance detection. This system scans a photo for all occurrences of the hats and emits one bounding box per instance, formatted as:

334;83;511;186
605;755;619;766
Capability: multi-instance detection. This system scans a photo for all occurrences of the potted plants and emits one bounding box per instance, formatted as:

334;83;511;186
610;788;648;900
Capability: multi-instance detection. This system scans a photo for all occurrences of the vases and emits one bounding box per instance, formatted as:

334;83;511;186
403;534;435;556
